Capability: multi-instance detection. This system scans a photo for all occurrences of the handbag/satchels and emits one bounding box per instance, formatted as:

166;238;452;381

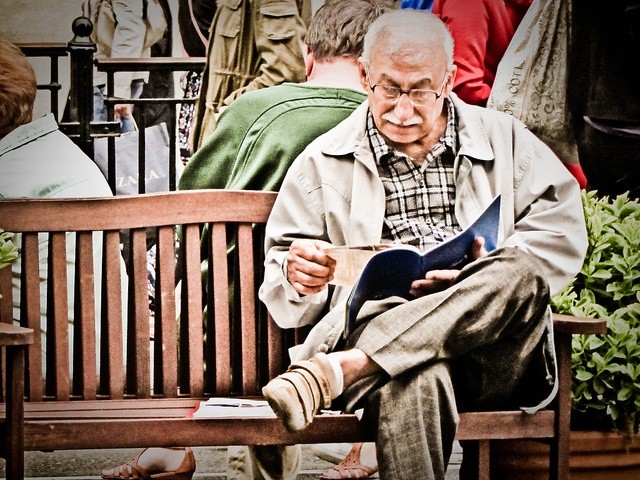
90;0;168;57
487;0;580;163
93;113;186;244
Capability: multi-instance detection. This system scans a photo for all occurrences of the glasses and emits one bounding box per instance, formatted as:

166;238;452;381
363;65;450;108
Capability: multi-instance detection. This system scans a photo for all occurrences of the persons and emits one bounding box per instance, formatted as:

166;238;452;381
560;0;640;197
130;0;397;476
102;0;377;480
431;1;587;186
2;36;133;395
258;9;590;480
62;0;168;137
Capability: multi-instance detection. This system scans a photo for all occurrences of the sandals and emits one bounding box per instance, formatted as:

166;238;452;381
319;442;380;480
101;446;195;480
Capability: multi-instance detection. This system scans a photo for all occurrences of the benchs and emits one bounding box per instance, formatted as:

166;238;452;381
1;190;608;479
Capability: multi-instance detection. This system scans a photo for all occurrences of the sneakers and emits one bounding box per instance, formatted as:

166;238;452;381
262;351;339;433
